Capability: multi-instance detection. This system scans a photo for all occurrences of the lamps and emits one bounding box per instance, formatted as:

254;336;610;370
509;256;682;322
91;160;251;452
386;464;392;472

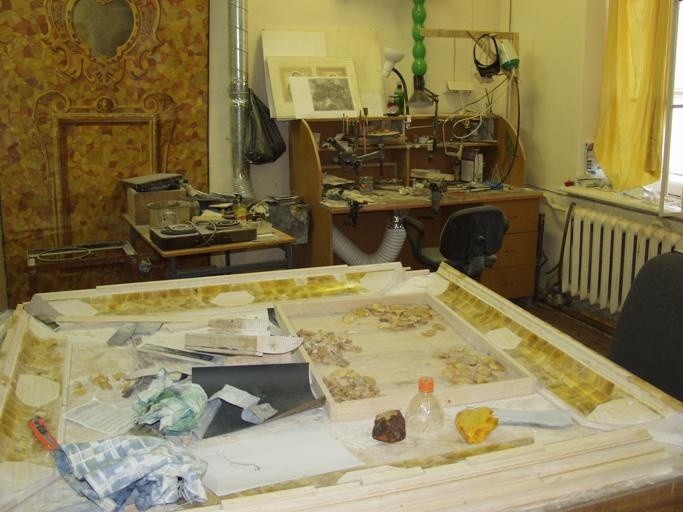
382;47;413;114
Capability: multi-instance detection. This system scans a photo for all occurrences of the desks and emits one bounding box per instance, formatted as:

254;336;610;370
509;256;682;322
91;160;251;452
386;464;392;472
124;214;296;280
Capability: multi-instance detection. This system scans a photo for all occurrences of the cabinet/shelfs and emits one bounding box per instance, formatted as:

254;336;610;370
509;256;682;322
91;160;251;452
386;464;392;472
290;114;543;307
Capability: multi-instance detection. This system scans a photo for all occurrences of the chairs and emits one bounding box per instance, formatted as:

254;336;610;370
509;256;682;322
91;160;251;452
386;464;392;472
418;205;508;284
612;251;683;405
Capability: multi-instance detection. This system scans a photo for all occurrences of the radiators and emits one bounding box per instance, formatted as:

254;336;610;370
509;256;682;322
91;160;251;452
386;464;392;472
559;208;683;324
